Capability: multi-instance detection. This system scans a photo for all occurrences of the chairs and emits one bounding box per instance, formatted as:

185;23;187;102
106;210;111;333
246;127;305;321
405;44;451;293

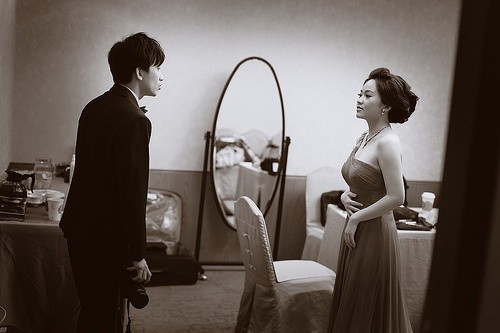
215;131;284;200
233;196;338;333
300;167;350;264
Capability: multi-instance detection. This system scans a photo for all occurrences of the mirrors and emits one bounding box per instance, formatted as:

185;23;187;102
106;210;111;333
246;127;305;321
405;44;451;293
210;56;286;231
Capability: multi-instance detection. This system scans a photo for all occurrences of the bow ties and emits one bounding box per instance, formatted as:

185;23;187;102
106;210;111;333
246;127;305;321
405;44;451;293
140;105;148;114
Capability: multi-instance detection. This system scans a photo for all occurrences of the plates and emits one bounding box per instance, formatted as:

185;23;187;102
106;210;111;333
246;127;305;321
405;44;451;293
27;189;64;200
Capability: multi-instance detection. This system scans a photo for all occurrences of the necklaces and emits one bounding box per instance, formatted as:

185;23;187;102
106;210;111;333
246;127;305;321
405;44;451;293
363;124;390;147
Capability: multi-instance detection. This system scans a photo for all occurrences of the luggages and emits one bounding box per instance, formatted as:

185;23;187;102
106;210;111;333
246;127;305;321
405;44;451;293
142;188;205;286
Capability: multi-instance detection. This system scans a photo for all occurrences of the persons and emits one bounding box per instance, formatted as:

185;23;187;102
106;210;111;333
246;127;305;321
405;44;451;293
58;32;165;333
325;68;420;333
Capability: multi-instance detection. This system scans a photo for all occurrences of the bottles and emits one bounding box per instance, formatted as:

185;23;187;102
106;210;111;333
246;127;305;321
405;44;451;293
70;154;75;184
32;155;54;189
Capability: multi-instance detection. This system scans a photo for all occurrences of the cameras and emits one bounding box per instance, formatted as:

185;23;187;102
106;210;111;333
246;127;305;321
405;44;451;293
119;268;148;309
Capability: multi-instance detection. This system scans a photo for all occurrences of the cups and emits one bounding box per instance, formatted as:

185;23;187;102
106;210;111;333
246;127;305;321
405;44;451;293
421;192;436;213
27;194;42;202
48;198;64;222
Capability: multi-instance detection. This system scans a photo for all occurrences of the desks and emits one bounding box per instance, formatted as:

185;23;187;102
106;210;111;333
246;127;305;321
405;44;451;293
0;176;82;333
237;161;278;218
316;202;436;333
